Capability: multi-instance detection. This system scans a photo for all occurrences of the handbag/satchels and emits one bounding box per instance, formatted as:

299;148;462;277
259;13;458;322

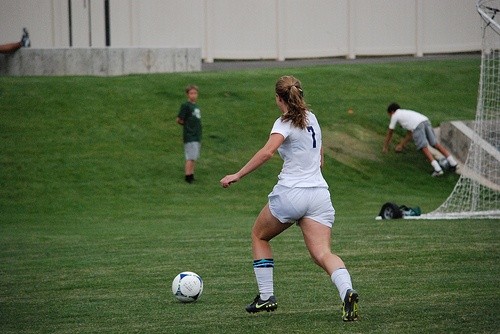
398;204;421;217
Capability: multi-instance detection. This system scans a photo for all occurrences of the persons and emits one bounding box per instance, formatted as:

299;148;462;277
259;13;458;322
382;102;460;177
220;74;359;321
0;26;31;54
176;84;203;184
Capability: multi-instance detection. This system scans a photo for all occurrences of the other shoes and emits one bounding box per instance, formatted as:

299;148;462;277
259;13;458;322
446;160;457;168
432;170;445;177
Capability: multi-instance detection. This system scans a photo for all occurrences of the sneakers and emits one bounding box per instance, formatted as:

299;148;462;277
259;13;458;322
341;289;359;321
246;294;277;314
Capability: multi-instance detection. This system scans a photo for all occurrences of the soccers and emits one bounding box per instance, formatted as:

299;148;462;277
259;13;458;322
171;271;204;303
